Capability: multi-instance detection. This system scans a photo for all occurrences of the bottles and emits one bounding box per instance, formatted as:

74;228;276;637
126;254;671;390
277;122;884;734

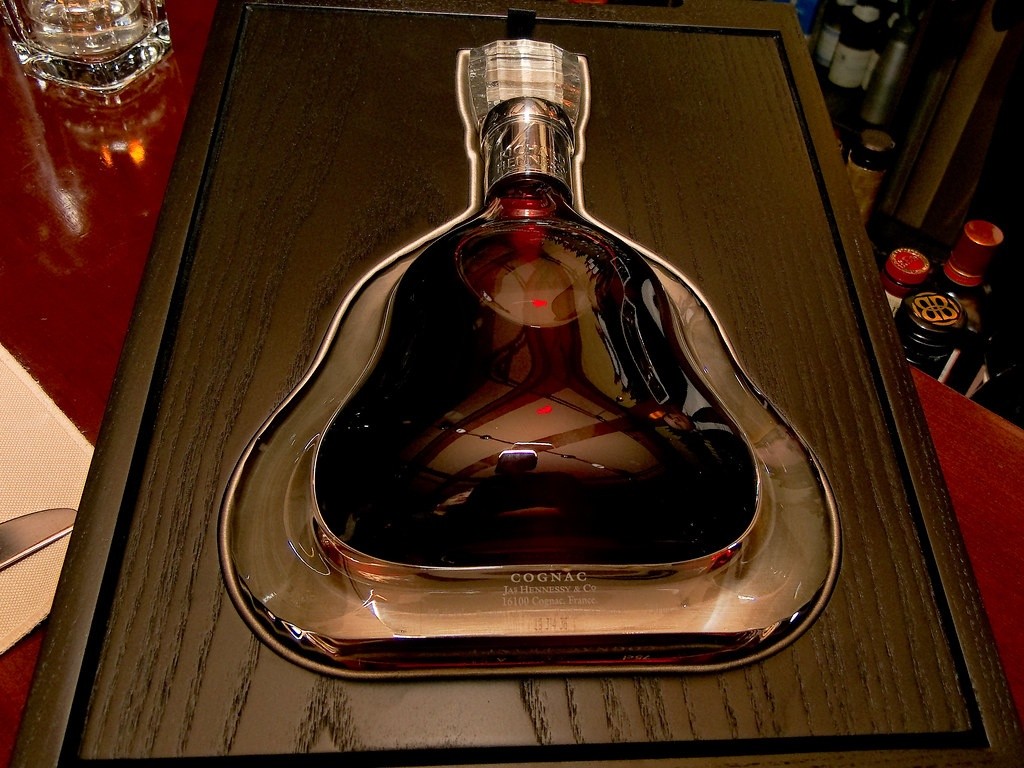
315;41;758;565
876;218;1004;419
792;0;934;232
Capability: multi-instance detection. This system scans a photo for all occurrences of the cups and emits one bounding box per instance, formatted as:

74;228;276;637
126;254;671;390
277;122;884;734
0;0;173;92
